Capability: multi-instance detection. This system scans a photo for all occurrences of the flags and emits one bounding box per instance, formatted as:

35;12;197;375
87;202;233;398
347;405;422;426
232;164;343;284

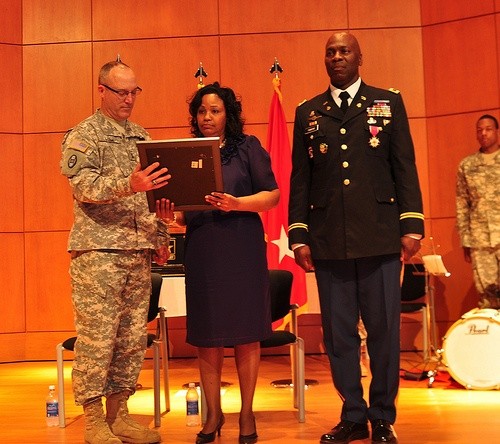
260;77;308;333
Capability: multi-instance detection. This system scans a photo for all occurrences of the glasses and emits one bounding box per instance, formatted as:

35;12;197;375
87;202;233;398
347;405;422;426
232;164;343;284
99;84;142;98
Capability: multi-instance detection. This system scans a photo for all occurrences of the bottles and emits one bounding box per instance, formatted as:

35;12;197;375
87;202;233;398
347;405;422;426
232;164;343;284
46;385;59;427
186;382;201;427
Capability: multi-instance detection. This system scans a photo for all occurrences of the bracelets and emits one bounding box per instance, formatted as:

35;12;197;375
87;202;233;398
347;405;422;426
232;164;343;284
160;211;177;226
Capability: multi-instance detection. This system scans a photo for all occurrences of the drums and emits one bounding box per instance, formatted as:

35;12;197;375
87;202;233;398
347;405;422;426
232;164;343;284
440;308;500;391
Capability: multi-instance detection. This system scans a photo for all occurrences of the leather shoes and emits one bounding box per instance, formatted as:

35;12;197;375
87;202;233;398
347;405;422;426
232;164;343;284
373;419;398;444
320;421;369;444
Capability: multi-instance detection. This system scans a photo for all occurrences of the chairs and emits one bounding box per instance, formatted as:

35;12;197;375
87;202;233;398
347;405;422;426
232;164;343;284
399;262;429;362
201;269;305;424
55;273;170;428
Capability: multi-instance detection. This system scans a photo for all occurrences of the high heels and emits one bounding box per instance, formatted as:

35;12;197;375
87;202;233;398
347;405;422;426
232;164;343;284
239;416;258;444
196;423;223;444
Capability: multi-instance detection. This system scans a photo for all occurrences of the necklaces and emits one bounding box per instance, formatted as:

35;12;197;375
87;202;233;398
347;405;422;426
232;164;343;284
219;136;226;149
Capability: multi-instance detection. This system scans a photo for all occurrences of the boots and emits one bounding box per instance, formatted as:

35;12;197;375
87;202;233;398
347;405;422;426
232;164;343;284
83;391;161;444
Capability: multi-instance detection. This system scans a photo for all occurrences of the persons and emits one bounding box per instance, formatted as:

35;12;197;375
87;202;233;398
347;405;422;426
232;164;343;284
59;60;171;444
153;81;280;444
455;114;500;310
287;31;425;444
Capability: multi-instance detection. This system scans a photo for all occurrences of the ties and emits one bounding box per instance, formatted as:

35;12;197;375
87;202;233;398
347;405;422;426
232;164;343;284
339;92;350;114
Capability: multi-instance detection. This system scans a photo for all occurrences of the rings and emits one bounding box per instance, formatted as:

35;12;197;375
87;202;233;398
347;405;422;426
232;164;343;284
153;180;156;185
216;202;222;206
